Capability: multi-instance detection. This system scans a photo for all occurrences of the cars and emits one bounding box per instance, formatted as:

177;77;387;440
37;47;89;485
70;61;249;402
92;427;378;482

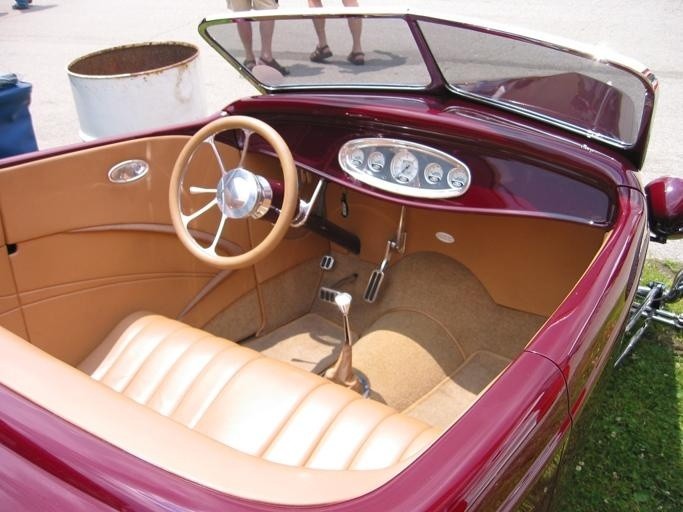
1;13;683;512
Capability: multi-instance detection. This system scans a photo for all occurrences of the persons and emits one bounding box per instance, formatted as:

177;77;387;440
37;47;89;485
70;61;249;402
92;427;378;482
307;0;366;65
224;0;290;78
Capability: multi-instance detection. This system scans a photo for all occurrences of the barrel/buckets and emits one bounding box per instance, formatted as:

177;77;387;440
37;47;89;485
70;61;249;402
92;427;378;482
66;39;200;143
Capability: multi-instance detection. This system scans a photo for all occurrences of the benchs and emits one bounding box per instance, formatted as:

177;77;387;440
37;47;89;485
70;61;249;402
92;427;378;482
1;307;450;507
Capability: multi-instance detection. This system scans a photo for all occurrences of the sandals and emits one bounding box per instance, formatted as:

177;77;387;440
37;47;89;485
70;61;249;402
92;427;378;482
258;58;289;75
311;45;333;60
348;51;364;64
243;59;256;71
